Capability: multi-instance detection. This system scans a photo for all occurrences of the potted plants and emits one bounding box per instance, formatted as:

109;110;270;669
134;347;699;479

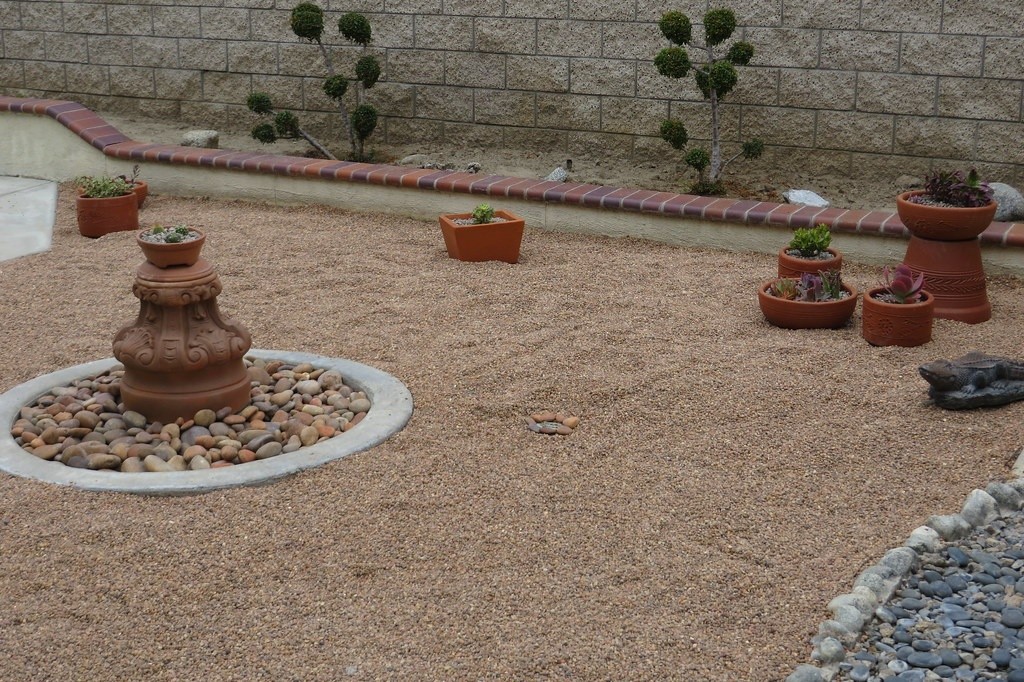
757;268;858;329
893;166;996;242
776;223;842;278
438;203;524;264
128;163;148;205
135;223;206;269
862;261;935;347
74;175;140;238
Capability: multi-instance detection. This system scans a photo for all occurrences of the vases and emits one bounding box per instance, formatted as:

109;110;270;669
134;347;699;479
902;233;991;323
